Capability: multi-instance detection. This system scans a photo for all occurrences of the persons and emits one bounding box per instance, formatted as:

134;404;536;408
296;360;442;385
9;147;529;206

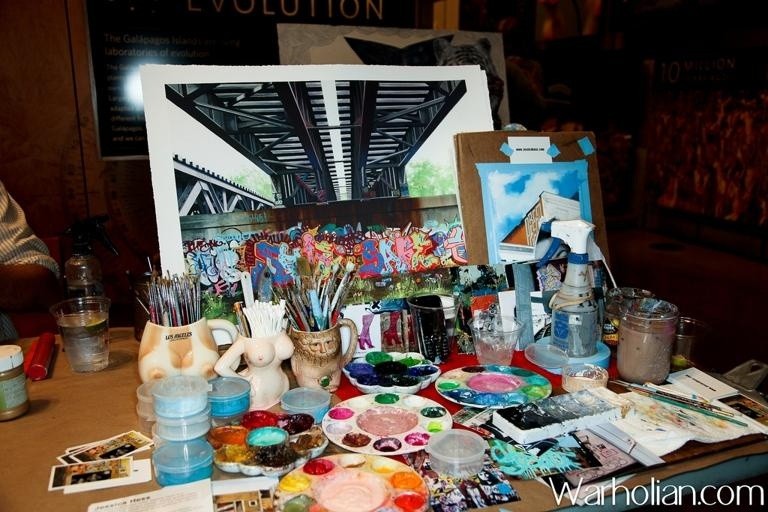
0;179;64;348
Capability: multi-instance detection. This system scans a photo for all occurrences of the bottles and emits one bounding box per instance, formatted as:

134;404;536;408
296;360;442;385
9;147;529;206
65;254;102;293
0;342;30;421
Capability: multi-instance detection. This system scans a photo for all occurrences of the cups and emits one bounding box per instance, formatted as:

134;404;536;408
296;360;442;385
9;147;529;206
137;288;703;512
49;293;112;374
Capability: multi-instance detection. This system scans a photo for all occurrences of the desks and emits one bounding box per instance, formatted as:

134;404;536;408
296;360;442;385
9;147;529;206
0;325;768;512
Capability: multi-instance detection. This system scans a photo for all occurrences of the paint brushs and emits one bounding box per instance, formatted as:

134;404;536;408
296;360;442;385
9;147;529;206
135;255;201;327
609;380;747;428
272;257;356;333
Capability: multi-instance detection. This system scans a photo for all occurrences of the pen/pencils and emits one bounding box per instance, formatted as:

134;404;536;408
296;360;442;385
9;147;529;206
233;302;251;340
23;341;39;382
